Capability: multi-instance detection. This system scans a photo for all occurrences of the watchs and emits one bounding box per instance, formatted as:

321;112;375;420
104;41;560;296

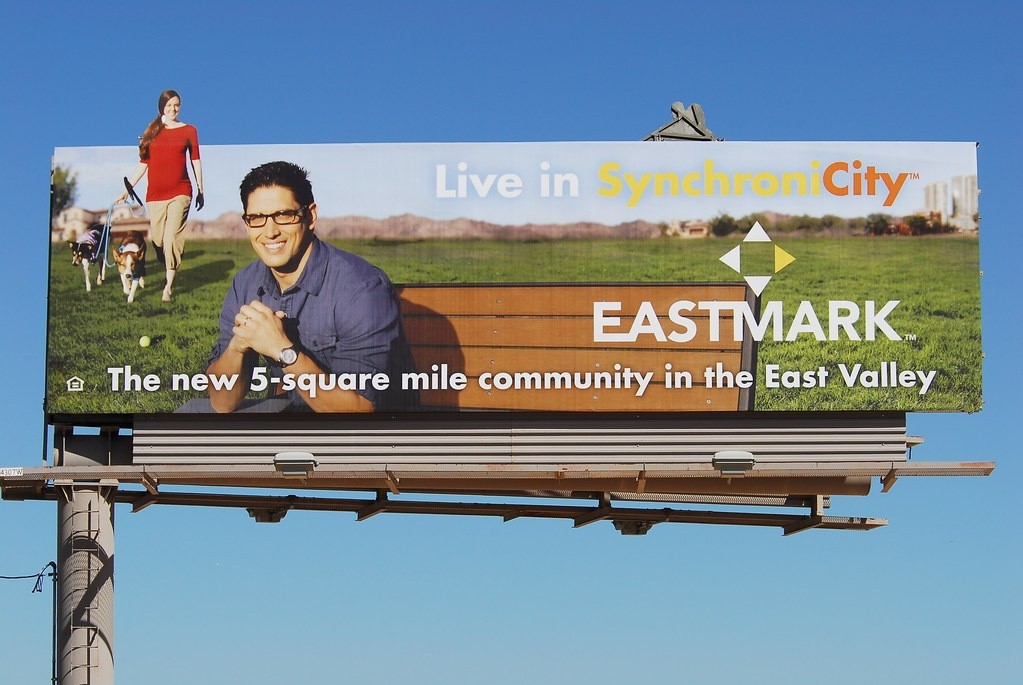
276;343;300;368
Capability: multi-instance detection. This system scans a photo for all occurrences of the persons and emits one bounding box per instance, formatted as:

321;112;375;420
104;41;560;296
114;90;204;301
173;161;418;413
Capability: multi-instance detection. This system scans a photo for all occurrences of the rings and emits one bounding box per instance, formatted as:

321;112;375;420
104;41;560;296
244;317;251;325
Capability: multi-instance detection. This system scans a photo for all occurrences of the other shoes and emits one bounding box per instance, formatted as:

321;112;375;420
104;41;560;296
162;288;172;301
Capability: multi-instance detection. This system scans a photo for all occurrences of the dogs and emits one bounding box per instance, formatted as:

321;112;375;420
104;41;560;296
66;224;111;294
112;230;147;303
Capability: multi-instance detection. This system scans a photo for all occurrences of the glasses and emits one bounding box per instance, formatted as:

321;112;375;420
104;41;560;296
241;204;309;228
